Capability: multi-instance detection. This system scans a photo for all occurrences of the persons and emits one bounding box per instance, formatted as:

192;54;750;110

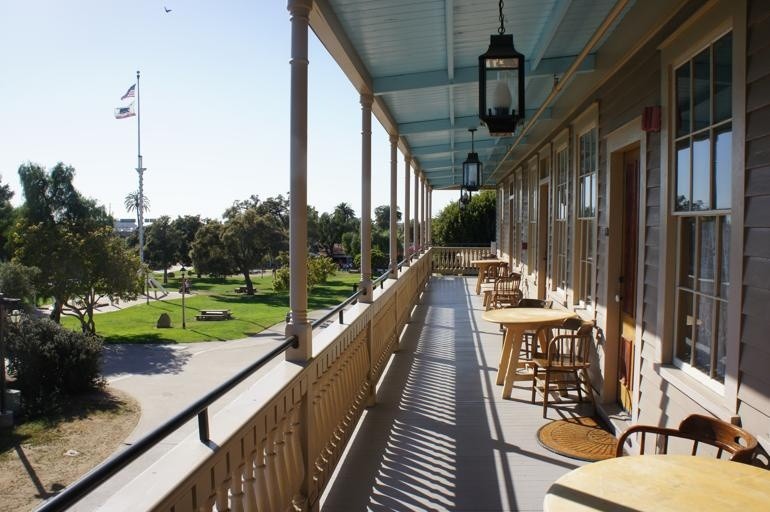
337;261;353;273
184;279;192;294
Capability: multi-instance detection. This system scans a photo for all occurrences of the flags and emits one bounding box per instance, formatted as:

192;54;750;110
112;81;137;120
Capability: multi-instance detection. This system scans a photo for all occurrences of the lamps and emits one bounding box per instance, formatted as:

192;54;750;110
458;1;527;213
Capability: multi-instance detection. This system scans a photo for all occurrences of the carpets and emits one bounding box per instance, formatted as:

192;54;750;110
535;416;630;461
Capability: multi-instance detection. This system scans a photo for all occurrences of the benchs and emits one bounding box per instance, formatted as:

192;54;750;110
195;312;233;321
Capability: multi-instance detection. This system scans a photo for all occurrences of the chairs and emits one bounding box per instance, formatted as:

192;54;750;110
481;257;597;419
616;413;759;465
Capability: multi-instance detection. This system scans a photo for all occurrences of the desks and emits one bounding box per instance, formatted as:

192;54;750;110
199;308;231;320
470;259;508;295
541;453;770;512
